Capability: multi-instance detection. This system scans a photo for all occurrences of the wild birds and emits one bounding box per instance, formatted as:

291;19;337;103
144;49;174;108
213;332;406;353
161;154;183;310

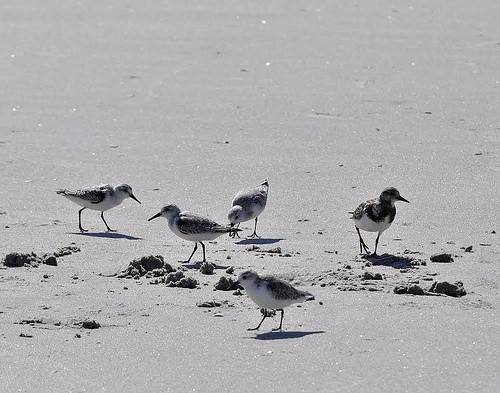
348;186;410;258
55;183;141;234
148;203;243;264
227;269;316;333
227;177;269;239
